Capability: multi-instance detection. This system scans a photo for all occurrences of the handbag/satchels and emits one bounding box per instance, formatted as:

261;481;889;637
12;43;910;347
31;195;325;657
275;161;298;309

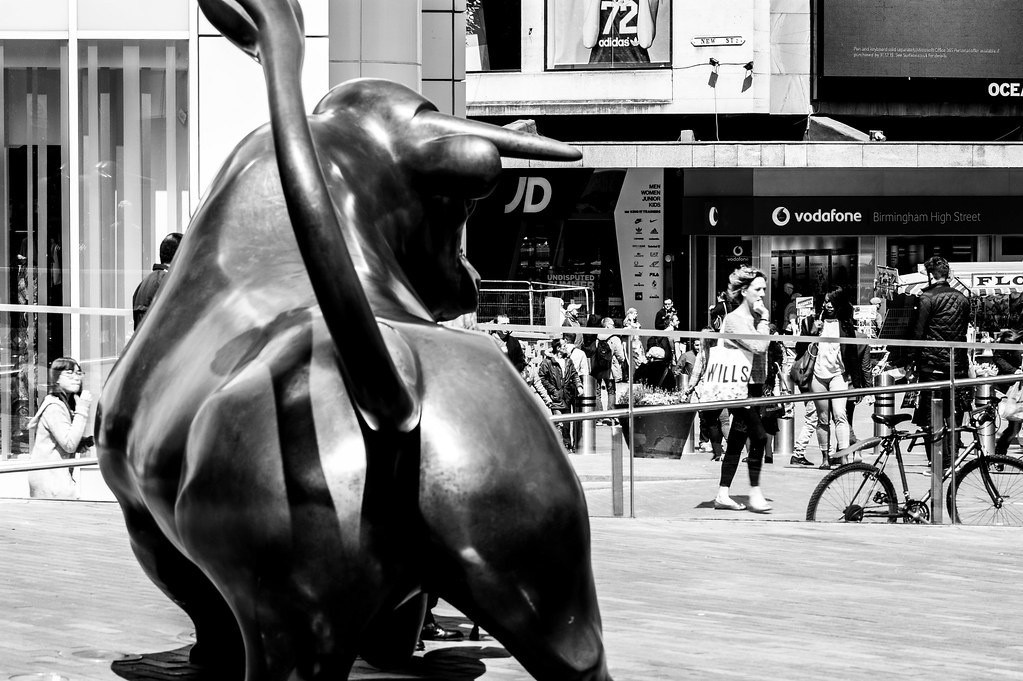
790;311;824;389
1003;381;1023;421
698;317;754;409
852;394;875;440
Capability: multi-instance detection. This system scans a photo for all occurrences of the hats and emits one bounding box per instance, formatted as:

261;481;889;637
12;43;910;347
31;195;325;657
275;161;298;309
567;304;582;312
646;346;665;359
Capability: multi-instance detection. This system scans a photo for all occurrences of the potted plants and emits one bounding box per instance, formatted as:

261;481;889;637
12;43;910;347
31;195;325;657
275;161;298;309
614;378;697;460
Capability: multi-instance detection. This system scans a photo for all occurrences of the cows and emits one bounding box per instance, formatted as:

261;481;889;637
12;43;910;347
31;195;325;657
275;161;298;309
95;0;615;681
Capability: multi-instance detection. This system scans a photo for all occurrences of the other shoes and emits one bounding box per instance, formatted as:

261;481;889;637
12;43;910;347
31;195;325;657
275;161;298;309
747;501;771;511
790;456;814;466
714;497;747;510
820;463;830;469
993;463;1004;471
923;469;932;476
742;457;747;461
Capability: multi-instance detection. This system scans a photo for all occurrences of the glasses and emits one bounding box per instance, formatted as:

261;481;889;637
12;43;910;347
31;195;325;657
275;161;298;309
60;371;85;378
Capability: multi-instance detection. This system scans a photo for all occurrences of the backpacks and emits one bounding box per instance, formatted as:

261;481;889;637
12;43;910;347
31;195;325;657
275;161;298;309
596;335;617;373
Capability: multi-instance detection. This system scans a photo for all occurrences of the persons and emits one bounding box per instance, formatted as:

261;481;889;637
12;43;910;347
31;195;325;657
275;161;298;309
594;299;683;425
490;304;589;452
583;0;658;64
133;233;183;332
28;358;94;498
992;332;1023;471
675;268;882;510
913;256;971;476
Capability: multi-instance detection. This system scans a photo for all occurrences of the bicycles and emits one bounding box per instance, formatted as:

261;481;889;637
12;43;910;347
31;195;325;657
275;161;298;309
805;388;1023;525
